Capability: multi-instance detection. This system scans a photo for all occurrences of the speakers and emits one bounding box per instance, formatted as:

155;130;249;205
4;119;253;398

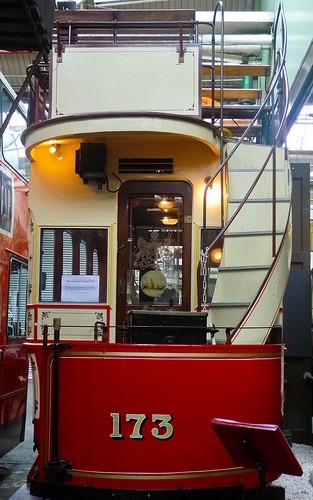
78;141;107;178
